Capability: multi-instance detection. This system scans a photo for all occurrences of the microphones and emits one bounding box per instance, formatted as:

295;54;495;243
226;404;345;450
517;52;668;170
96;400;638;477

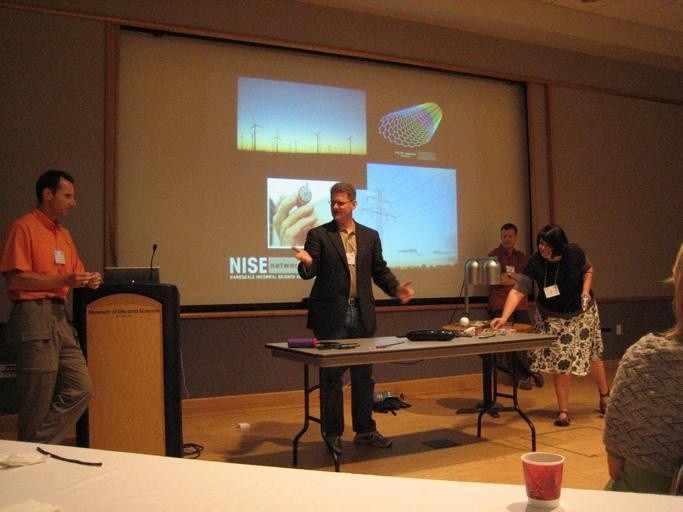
149;243;157;268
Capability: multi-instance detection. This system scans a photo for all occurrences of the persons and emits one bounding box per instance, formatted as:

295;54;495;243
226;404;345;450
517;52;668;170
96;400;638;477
486;223;545;390
288;182;413;454
0;169;103;445
491;224;611;425
267;191;318;246
603;242;683;495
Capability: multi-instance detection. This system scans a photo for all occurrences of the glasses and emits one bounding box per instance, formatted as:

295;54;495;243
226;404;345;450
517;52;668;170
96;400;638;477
328;199;353;205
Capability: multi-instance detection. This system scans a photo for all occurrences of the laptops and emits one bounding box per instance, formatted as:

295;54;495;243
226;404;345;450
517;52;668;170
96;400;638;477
103;267;160;284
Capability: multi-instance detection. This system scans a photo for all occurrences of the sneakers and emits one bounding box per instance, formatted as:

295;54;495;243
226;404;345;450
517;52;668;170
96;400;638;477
326;435;342;455
517;376;532;390
532;373;544;387
353;430;392;448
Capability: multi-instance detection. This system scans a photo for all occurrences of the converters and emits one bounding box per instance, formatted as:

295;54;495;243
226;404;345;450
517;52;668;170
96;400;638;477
237;422;250;429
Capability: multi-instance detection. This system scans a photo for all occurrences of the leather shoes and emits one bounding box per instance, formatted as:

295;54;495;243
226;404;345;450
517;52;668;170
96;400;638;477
554;410;571;426
599;389;611;414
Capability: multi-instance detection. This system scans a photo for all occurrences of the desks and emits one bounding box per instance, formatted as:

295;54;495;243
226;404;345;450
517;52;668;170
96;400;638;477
265;326;558;472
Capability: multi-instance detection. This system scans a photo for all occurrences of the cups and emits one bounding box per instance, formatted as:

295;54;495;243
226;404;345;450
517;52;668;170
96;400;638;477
521;450;564;510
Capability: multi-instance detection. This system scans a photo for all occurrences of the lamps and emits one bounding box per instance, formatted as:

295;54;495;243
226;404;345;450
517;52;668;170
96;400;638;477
464;257;502;326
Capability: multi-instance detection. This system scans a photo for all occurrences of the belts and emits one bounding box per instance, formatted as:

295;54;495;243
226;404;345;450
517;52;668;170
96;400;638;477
36;298;64;305
348;297;359;306
